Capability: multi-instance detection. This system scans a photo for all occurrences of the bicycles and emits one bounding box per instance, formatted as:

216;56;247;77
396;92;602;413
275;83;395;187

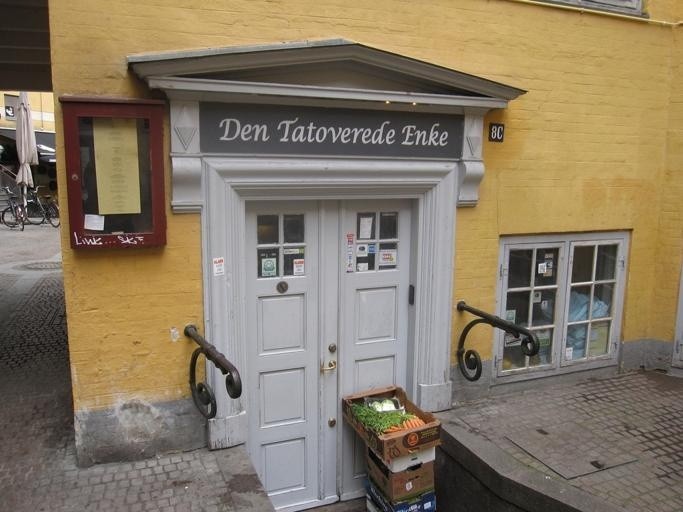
2;186;25;232
18;181;60;228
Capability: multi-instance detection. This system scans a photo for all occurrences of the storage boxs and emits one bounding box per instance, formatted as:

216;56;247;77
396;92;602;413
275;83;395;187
589;320;609;355
342;386;441;512
505;321;550;366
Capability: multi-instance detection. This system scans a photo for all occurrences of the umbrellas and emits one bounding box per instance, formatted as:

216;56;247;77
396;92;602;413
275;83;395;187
15;91;39;216
